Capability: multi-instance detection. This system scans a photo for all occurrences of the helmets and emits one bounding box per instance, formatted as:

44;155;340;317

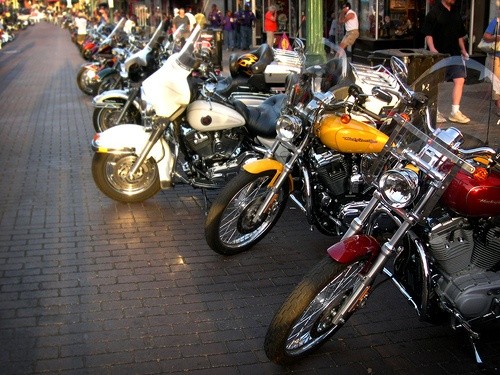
245;3;251;7
236;53;258;76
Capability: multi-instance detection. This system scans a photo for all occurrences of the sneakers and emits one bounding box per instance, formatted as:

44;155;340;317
449;112;470;123
436;114;447;123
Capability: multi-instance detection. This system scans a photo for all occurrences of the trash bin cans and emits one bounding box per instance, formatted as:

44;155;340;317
372;49;438;143
203;28;223;71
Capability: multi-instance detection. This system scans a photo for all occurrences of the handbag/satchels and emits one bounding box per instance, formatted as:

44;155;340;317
477;17;500;54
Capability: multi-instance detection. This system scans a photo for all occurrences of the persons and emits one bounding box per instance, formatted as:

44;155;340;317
208;1;257;52
333;2;360;64
326;12;336;55
483;0;500;117
421;0;470;123
171;7;207;40
264;5;279;51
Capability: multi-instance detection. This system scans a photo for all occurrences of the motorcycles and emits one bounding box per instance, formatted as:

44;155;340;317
203;36;485;256
63;0;414;204
264;55;500;369
